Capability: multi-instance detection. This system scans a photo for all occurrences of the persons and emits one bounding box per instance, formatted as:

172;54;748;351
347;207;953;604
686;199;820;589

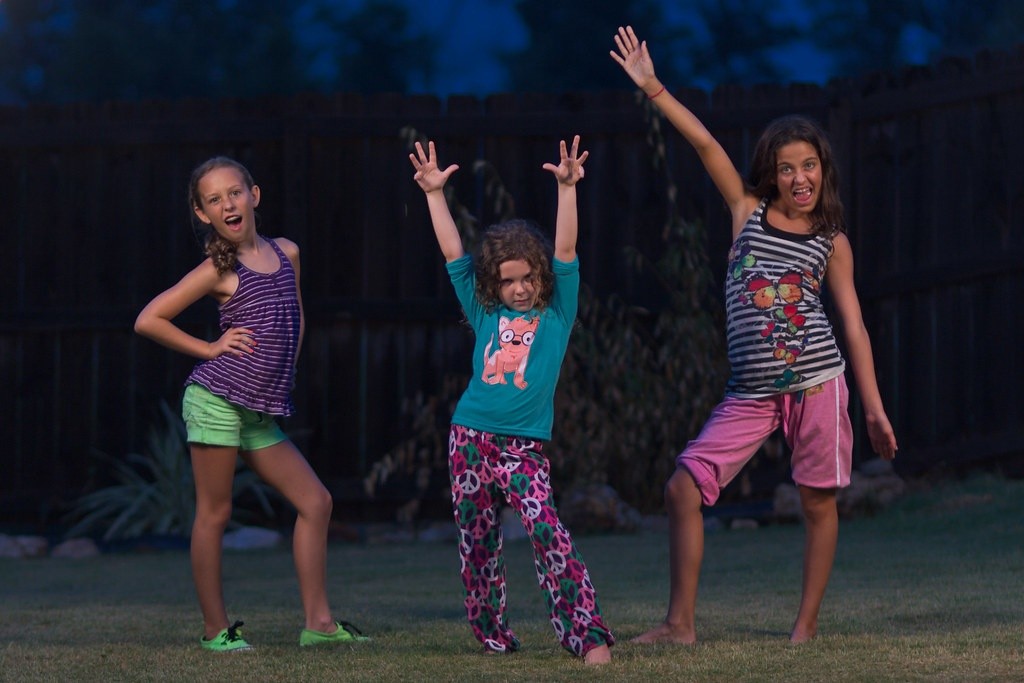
409;135;616;664
134;157;371;652
609;26;899;643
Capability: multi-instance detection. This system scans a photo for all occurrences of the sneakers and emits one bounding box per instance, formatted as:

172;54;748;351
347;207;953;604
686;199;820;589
299;618;373;649
200;620;256;654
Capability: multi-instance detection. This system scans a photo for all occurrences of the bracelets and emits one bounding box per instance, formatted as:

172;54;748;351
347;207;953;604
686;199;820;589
648;86;665;101
